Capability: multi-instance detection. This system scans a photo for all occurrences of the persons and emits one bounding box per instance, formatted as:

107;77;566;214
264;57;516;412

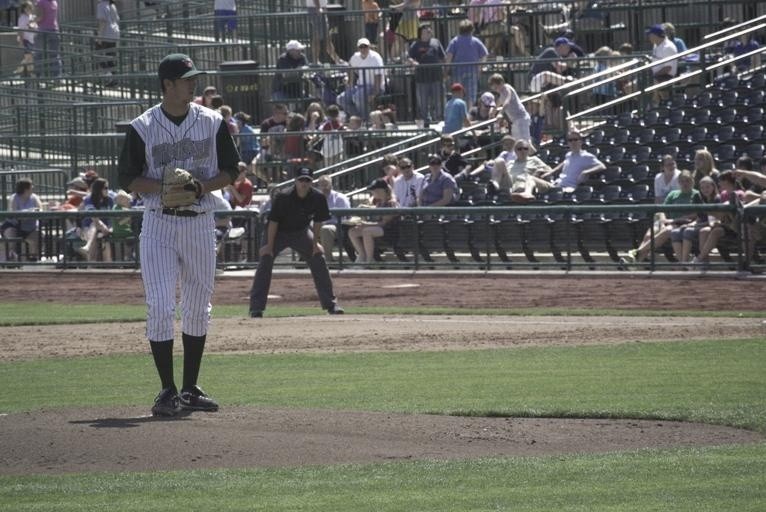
212;1;237;43
5;170;143;268
195;87;294;263
248;168;344;317
4;1;122;88
620;150;764;272
593;14;763;115
270;1;607;269
117;52;241;418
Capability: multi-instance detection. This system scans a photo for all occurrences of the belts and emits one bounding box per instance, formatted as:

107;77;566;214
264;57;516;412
150;208;205;216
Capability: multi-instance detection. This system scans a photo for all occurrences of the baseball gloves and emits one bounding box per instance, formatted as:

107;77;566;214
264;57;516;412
162;167;204;208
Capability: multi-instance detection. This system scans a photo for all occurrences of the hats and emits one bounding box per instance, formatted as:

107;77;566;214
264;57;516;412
356;37;369;47
451;83;466;97
481;93;496;107
65;176;88;189
158;53;207;79
554;36;574;47
285;39;304;51
645;24;664;38
366;178;388;190
296;167;313;181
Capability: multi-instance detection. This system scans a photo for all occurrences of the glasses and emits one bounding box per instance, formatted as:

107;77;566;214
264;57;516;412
298;177;310;182
398;135;580;170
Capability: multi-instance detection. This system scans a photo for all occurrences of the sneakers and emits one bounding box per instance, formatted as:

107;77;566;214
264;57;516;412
616;250;707;272
177;388;219;411
328;306;344;314
151;388;181;417
251;308;261;317
